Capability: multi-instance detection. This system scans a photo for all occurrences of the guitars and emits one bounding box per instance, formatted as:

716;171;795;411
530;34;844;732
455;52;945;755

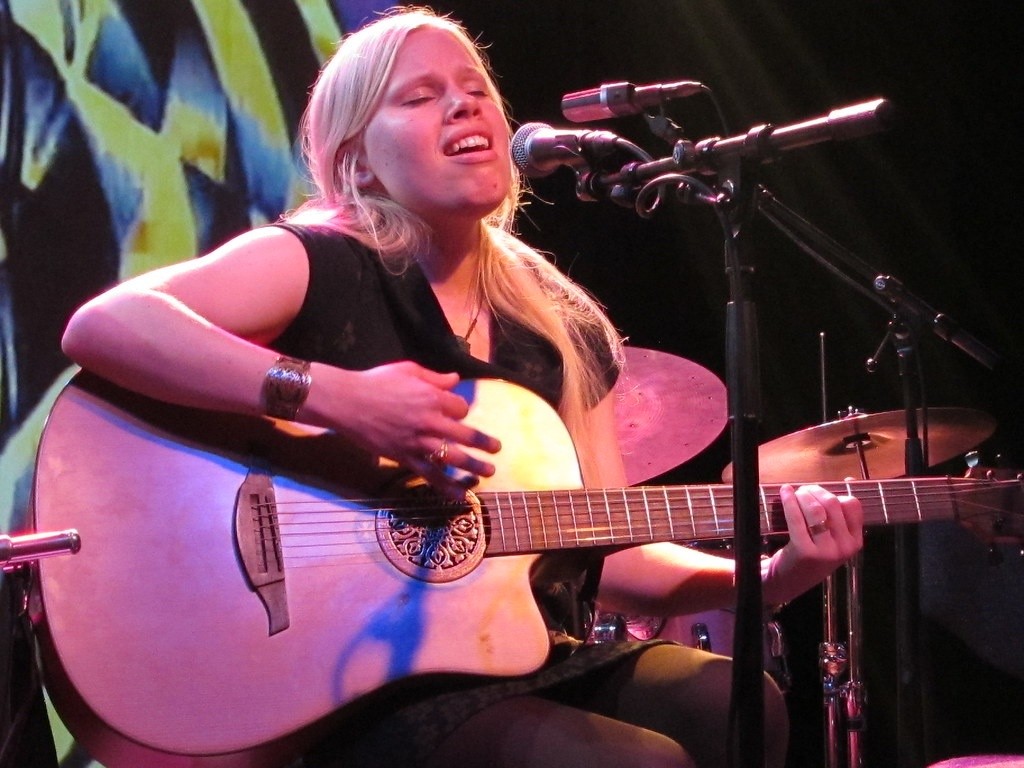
22;342;1023;768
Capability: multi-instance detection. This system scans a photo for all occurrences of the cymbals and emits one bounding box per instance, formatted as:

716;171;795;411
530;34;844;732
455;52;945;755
610;345;729;487
720;406;998;485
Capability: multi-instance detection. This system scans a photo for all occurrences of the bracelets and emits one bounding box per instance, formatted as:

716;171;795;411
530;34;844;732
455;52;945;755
265;354;312;422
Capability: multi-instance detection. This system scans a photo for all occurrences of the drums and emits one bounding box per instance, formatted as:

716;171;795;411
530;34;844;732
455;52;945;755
585;535;794;703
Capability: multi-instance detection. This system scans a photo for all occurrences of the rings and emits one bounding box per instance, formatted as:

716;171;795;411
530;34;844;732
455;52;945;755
808;519;830;536
424;439;449;468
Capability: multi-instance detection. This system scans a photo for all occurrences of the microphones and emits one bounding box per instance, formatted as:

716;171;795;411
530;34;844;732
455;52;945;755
509;122;620;180
561;82;706;123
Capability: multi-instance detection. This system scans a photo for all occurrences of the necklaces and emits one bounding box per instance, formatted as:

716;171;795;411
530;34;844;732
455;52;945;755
455;300;483;355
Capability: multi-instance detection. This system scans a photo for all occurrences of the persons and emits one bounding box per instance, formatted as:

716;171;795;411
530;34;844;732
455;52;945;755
61;11;863;768
